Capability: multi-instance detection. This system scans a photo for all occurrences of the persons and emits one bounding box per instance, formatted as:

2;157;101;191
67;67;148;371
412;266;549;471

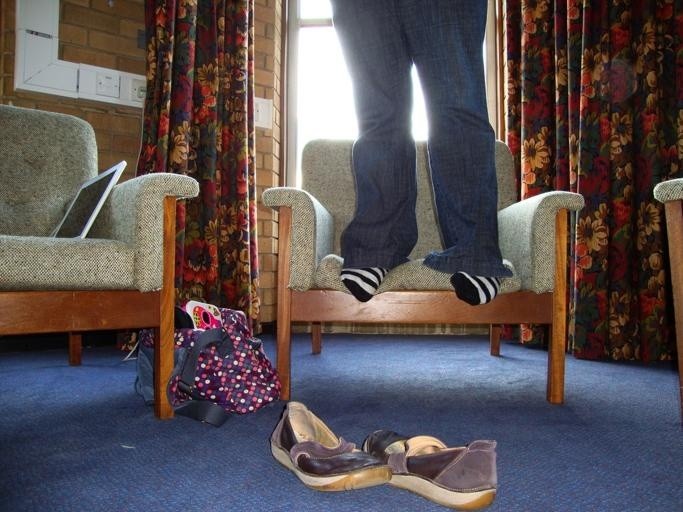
326;0;516;306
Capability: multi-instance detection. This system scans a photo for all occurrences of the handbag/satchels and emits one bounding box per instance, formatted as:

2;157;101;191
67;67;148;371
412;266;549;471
133;299;282;428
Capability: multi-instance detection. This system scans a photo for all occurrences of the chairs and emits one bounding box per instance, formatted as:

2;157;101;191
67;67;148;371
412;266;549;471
0;103;199;419
261;138;584;406
654;178;683;418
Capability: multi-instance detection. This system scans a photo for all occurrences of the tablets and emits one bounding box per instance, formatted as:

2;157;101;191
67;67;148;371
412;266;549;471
54;159;128;239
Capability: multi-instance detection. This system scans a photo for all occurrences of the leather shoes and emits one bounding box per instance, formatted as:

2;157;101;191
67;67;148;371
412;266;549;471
269;401;498;509
